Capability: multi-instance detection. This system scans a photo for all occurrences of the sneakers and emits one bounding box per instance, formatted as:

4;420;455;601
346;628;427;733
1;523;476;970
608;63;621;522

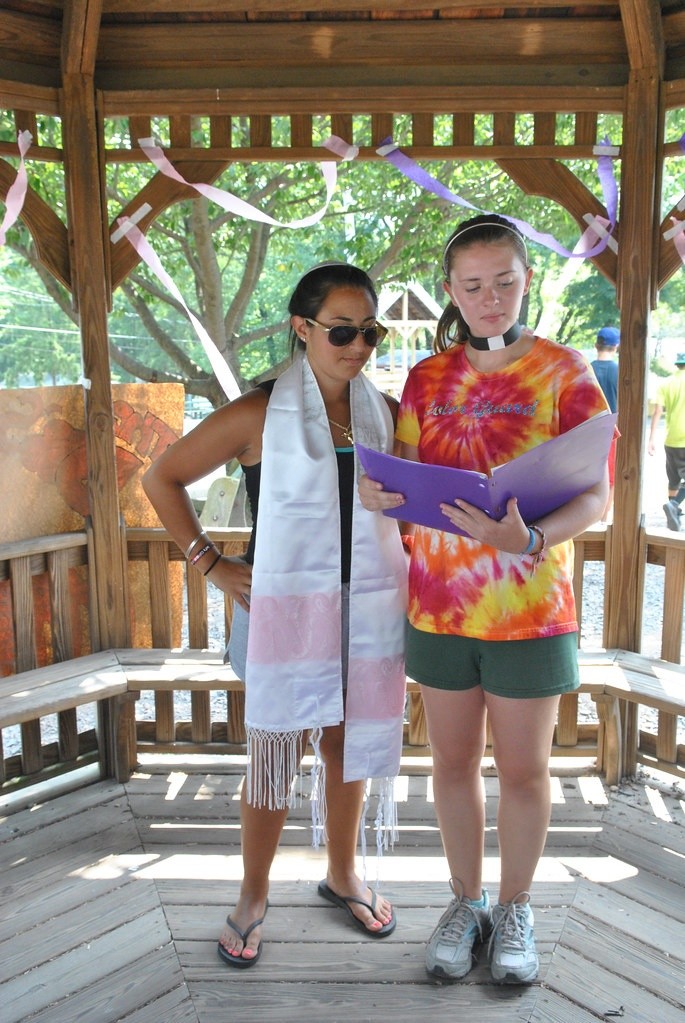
425;876;495;979
489;890;539;984
663;502;685;531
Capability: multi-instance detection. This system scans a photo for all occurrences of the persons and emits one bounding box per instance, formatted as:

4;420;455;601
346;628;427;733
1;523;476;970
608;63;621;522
358;210;610;986
647;351;685;531
140;259;400;964
590;327;619;520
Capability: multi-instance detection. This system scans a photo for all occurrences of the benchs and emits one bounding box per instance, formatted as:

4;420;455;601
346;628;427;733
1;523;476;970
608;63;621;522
0;648;685;796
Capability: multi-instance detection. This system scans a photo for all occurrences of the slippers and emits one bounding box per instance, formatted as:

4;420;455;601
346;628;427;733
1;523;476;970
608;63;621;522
318;876;397;938
217;897;269;968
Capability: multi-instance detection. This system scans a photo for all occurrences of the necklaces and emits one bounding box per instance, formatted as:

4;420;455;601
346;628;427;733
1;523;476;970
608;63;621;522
327;418;353;447
469;320;521;351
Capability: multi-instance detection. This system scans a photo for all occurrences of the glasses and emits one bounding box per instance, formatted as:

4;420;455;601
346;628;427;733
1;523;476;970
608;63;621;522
304;318;388;347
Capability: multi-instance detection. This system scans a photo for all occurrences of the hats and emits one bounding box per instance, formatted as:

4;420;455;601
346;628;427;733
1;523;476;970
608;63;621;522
597;327;621;346
674;353;685;365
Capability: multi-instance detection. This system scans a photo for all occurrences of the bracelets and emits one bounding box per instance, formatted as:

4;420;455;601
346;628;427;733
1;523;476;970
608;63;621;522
528;525;546;556
184;530;206;558
203;553;222;575
521;528;536;556
189;541;216;565
